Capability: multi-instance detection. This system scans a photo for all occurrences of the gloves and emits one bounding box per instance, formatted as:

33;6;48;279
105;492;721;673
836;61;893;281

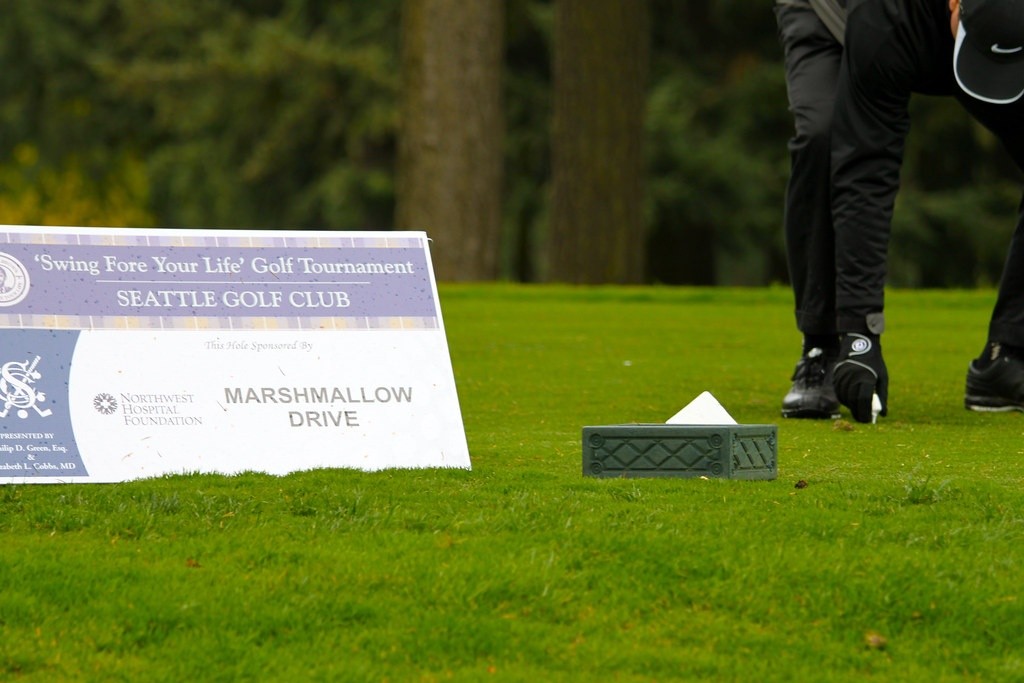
835;320;889;423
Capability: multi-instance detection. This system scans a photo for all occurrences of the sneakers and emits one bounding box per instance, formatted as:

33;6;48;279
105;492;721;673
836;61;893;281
964;356;1023;413
782;338;842;418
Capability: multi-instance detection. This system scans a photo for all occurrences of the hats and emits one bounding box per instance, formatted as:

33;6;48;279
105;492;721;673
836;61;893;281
953;0;1024;104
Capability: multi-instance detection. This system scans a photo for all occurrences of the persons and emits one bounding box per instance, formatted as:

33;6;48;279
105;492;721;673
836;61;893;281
775;0;1024;428
